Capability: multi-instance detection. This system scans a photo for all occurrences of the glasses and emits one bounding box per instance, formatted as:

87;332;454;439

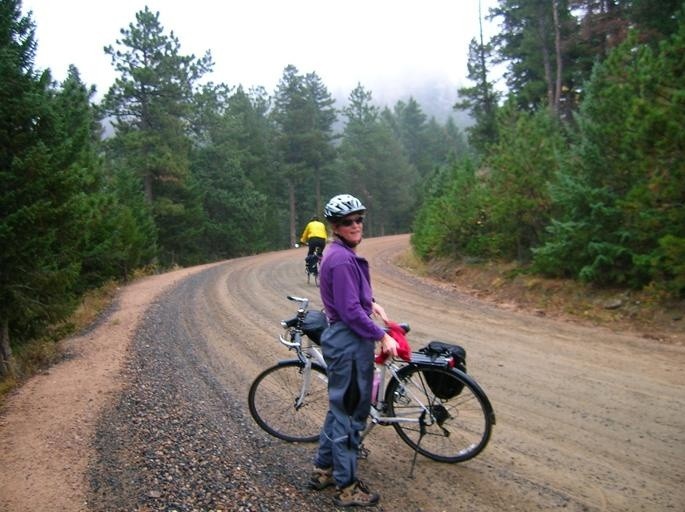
336;217;363;227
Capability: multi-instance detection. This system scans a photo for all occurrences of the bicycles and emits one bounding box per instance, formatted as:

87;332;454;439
296;241;323;287
248;296;497;479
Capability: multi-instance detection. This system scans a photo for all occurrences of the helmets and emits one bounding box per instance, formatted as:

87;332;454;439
324;194;366;218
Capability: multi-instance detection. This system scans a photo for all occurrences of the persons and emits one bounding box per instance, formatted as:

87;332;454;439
299;215;327;275
309;193;401;507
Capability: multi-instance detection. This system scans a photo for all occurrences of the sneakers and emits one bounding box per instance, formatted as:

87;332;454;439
310;466;337;490
333;478;381;507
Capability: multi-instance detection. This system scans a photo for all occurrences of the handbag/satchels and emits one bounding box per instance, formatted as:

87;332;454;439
305;255;317;273
302;308;328;345
419;342;466;399
